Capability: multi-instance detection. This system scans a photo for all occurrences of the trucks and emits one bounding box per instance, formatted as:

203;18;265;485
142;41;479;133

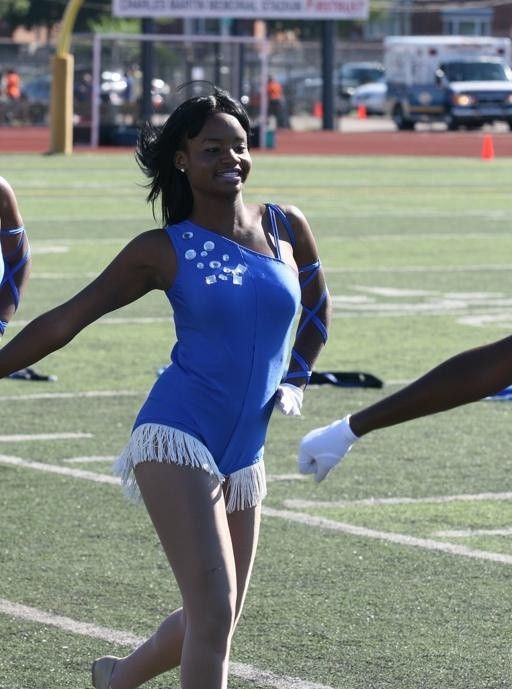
283;32;512;130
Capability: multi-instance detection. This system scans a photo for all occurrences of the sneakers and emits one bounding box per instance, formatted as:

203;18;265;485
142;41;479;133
92;656;115;688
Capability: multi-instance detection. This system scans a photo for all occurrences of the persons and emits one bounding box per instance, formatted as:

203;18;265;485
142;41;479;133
0;176;30;336
0;90;331;688
299;335;512;483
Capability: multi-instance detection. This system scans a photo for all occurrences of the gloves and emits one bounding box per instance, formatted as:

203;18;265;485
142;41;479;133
276;383;303;417
298;413;360;483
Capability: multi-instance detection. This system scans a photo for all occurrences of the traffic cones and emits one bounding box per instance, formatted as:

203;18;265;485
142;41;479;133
481;133;495;160
